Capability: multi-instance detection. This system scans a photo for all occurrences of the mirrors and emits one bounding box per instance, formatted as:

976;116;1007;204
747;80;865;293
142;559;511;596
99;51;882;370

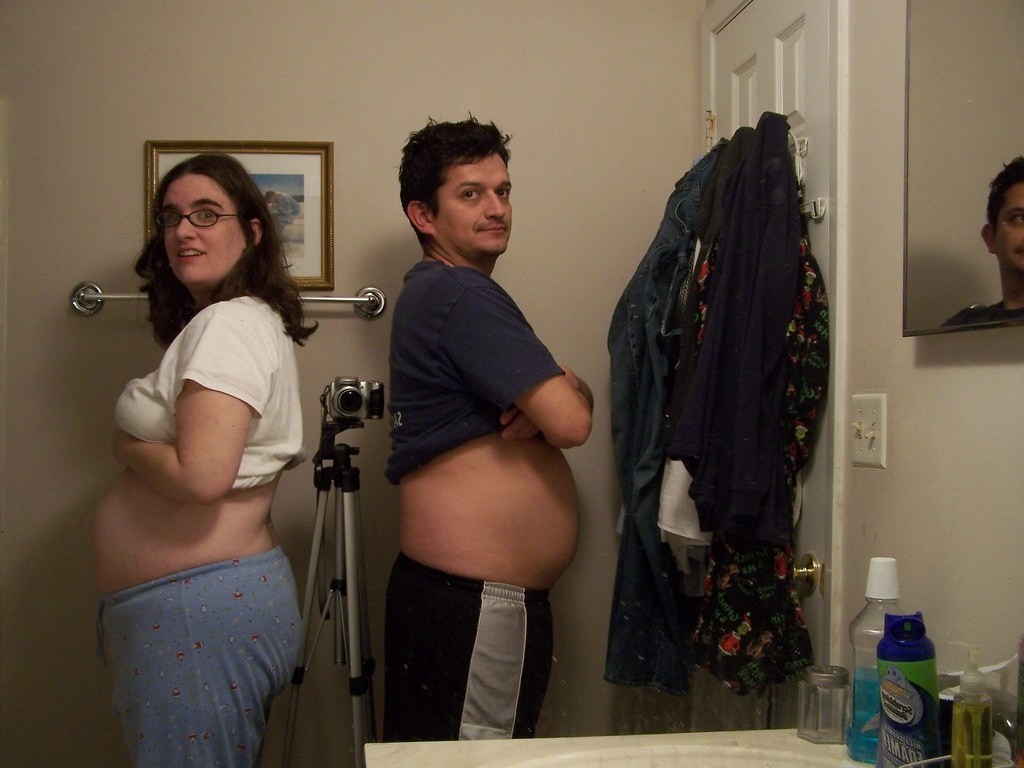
902;0;1024;338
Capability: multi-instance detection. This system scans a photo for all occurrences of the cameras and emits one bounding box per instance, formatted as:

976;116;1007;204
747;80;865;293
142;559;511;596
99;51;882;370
329;376;385;421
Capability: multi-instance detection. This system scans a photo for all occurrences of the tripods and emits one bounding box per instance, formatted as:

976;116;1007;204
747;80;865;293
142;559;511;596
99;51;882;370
281;420;377;768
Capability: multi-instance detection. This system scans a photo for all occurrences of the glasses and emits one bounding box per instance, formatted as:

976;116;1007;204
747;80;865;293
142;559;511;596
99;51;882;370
153;209;241;228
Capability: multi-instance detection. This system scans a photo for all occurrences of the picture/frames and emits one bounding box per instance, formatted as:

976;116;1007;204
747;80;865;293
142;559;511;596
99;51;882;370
144;139;335;289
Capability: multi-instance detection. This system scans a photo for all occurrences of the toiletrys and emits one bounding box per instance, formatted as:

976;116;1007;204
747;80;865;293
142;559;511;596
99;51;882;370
946;640;1023;768
847;557;906;765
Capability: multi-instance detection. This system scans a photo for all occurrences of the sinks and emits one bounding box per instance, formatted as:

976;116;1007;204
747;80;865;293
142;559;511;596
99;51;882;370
519;745;854;768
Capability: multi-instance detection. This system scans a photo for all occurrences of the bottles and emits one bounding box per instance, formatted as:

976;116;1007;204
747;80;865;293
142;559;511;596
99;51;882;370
797;664;852;745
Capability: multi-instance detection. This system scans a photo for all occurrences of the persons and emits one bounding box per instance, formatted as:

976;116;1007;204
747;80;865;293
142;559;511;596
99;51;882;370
942;150;1024;330
88;153;305;768
378;113;594;740
